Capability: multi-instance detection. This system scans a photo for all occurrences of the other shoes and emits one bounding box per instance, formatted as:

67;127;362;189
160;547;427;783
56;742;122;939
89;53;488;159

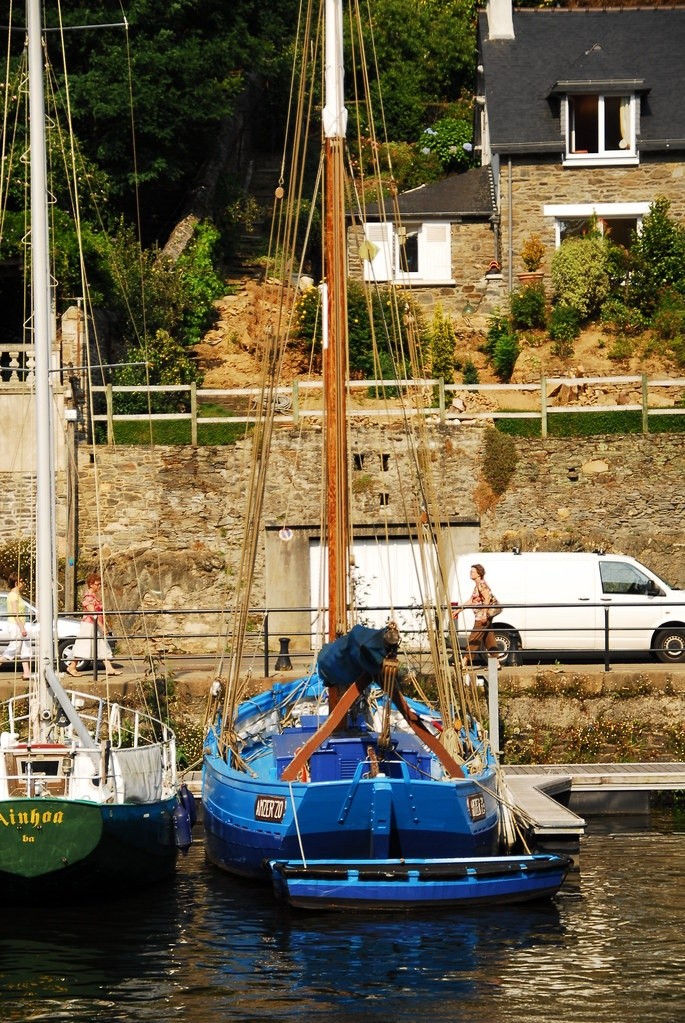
22;672;35;680
66;667;123;679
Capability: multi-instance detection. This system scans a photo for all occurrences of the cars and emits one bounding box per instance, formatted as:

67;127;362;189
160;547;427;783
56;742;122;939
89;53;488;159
0;590;93;672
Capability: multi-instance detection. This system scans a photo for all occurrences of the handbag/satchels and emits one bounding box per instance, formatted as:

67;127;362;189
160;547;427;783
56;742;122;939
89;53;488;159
487;601;502;617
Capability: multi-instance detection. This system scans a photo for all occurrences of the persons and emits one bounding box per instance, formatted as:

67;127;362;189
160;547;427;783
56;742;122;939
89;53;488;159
0;572;36;680
66;574;123;677
454;564;503;670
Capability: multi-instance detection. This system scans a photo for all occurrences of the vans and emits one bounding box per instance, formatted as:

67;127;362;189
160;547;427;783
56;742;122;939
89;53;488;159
442;552;685;666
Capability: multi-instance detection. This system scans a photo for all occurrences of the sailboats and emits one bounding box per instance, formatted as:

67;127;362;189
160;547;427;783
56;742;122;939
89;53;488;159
185;3;573;917
2;0;185;909
